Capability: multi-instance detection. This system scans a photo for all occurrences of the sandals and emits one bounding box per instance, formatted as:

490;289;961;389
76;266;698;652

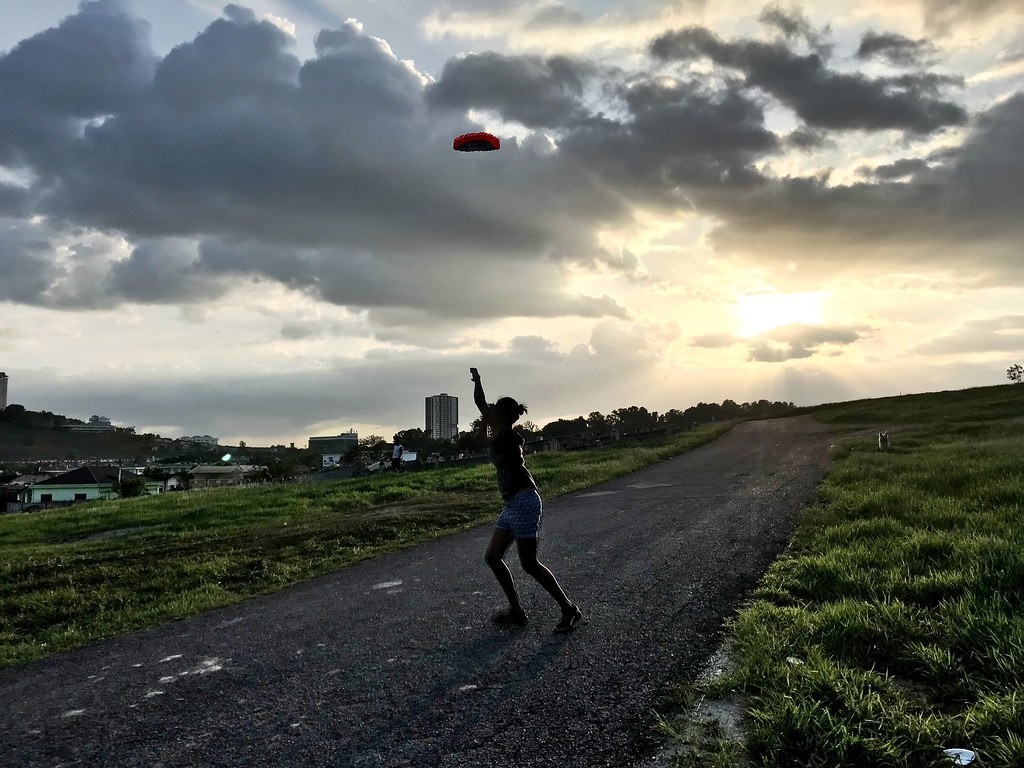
491;609;529;627
554;604;582;634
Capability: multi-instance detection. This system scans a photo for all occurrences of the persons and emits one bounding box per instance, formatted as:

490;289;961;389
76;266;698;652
391;439;402;473
469;367;581;632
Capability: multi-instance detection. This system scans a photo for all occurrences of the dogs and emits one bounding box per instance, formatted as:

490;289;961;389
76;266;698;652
879;433;889;449
